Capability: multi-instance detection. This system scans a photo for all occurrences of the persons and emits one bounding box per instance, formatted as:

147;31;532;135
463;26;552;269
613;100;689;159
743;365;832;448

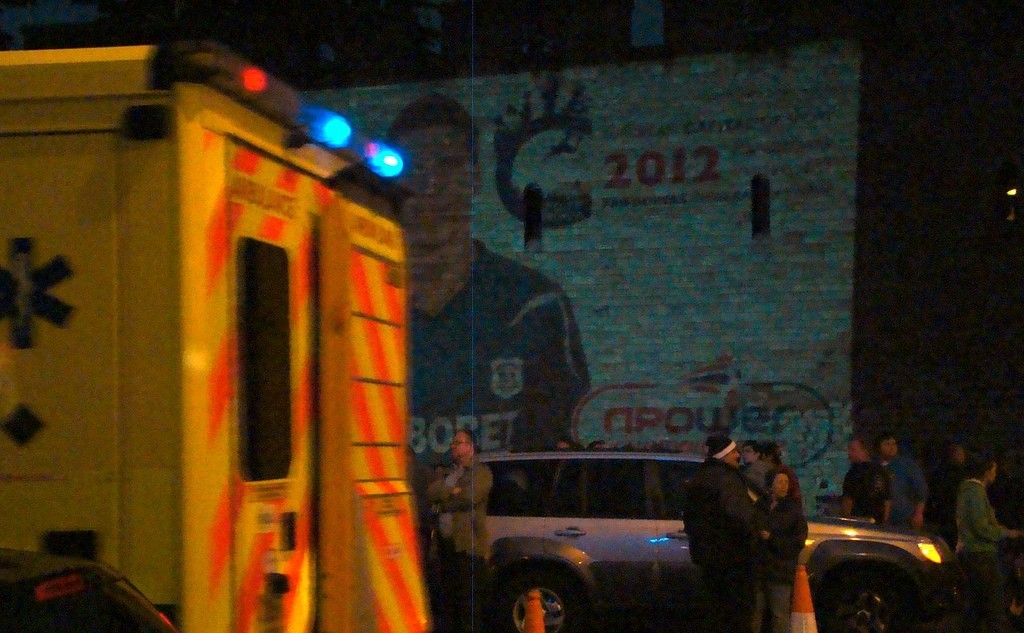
841;437;893;526
874;435;1024;633
387;96;589;465
427;430;493;633
954;456;1024;633
406;445;443;572
683;433;808;633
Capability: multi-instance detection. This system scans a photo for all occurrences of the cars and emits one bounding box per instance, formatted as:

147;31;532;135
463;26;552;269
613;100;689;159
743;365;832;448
0;548;180;633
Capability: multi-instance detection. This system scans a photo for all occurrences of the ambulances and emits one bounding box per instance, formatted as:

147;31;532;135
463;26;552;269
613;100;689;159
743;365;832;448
0;38;434;633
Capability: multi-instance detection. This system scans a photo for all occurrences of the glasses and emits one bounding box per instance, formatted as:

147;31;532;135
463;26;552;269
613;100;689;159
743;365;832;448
450;440;468;445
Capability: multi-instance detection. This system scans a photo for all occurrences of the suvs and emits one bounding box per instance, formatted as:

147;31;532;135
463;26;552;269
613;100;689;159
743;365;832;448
474;452;968;633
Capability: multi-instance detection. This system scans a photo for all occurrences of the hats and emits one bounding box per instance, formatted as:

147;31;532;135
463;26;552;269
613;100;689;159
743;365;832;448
705;435;737;459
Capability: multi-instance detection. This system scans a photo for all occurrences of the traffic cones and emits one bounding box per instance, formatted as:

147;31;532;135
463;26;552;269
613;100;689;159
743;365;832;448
525;589;546;633
790;564;817;633
